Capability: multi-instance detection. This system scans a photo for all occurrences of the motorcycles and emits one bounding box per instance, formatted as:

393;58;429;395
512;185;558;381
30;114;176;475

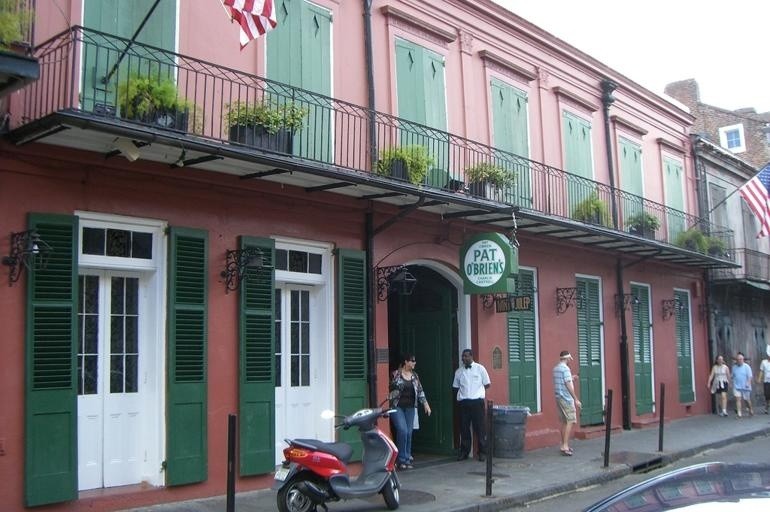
273;389;401;512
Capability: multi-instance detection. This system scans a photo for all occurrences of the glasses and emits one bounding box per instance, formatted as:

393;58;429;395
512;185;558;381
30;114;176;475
408;360;415;362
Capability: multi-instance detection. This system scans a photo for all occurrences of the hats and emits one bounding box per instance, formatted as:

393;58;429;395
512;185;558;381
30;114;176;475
560;353;573;360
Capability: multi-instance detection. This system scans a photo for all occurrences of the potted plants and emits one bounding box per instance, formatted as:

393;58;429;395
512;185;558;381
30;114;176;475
371;144;515;200
572;191;725;256
115;58;313;158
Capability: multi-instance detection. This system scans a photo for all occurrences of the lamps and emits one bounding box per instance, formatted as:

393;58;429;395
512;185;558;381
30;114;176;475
1;228;52;287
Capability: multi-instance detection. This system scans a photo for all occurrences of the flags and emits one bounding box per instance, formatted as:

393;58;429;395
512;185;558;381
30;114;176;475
217;0;280;51
737;161;770;239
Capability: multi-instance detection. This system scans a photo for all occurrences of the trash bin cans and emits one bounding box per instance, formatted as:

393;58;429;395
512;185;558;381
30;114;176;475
492;405;531;458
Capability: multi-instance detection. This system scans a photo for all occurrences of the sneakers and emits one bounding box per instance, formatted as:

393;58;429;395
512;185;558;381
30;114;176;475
719;412;728;417
457;455;467;461
407;465;414;468
401;464;407;469
561;448;573;456
479;454;485;461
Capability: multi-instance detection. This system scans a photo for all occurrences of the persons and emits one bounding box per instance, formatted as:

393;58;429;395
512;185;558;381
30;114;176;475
452;349;491;461
706;354;732;416
757;344;770;414
552;349;584;456
729;351;754;420
386;350;432;470
